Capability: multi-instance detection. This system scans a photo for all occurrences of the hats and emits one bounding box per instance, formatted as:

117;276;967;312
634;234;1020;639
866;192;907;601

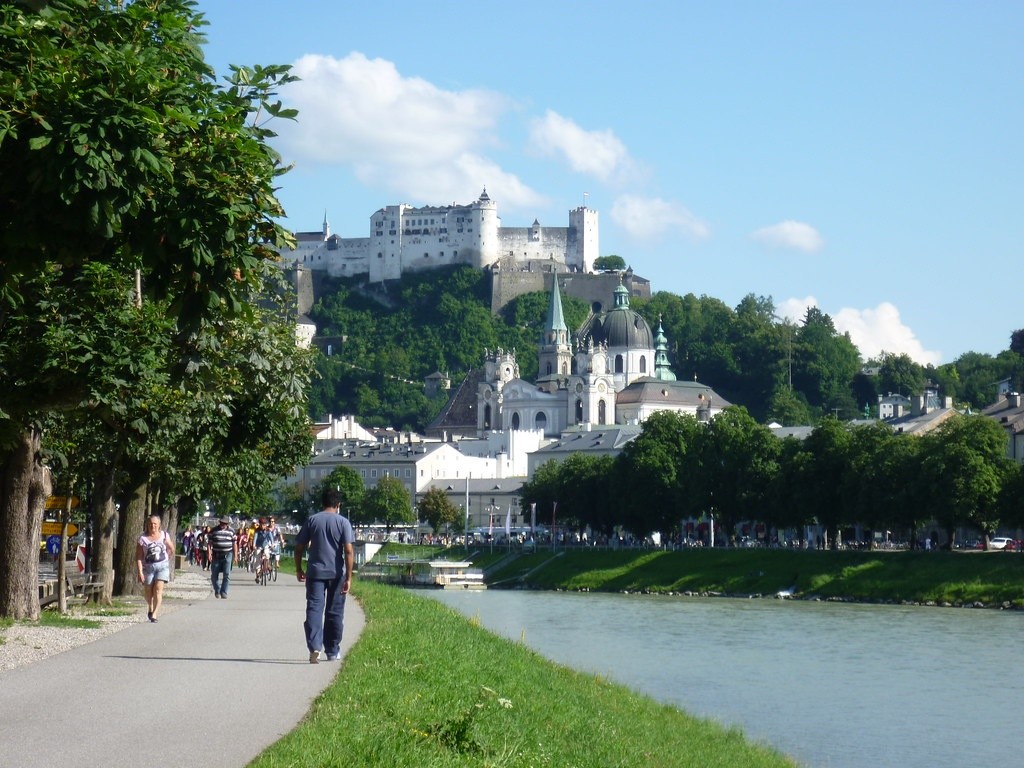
219;515;233;525
259;517;267;524
251;518;257;523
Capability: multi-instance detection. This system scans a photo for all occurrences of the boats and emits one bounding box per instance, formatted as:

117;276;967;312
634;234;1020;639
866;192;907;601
410;551;483;587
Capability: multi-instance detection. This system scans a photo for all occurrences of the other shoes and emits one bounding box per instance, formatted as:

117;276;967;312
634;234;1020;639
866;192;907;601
207;568;211;571
148;611;154;620
215;592;221;598
203;566;207;571
266;565;272;572
328;652;340;660
255;575;260;584
310;651;321;664
275;562;280;568
151;618;158;623
220;591;227;599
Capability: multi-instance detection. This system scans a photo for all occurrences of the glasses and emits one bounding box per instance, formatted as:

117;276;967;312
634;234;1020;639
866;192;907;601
270;521;274;522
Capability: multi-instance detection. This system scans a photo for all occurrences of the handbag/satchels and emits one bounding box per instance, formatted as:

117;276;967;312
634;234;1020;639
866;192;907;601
164;531;174;560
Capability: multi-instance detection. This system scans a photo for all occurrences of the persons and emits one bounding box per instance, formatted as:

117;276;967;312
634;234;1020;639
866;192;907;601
180;515;287;600
284;522;301;536
351;528;931;551
295;489;356;664
1002;537;1024;552
136;514;175;624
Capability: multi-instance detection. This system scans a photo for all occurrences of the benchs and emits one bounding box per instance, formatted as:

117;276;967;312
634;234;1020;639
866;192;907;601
39;572;105;605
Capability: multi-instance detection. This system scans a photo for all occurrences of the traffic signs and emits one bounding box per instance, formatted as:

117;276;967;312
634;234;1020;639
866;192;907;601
45;496;79;509
42;522;77;537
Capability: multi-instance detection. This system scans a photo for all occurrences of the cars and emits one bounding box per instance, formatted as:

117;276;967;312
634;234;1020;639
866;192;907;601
965;539;979;549
990;537;1014;550
975;542;986;550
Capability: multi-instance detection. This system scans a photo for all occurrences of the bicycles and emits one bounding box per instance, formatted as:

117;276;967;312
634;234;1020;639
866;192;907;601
238;543;284;587
